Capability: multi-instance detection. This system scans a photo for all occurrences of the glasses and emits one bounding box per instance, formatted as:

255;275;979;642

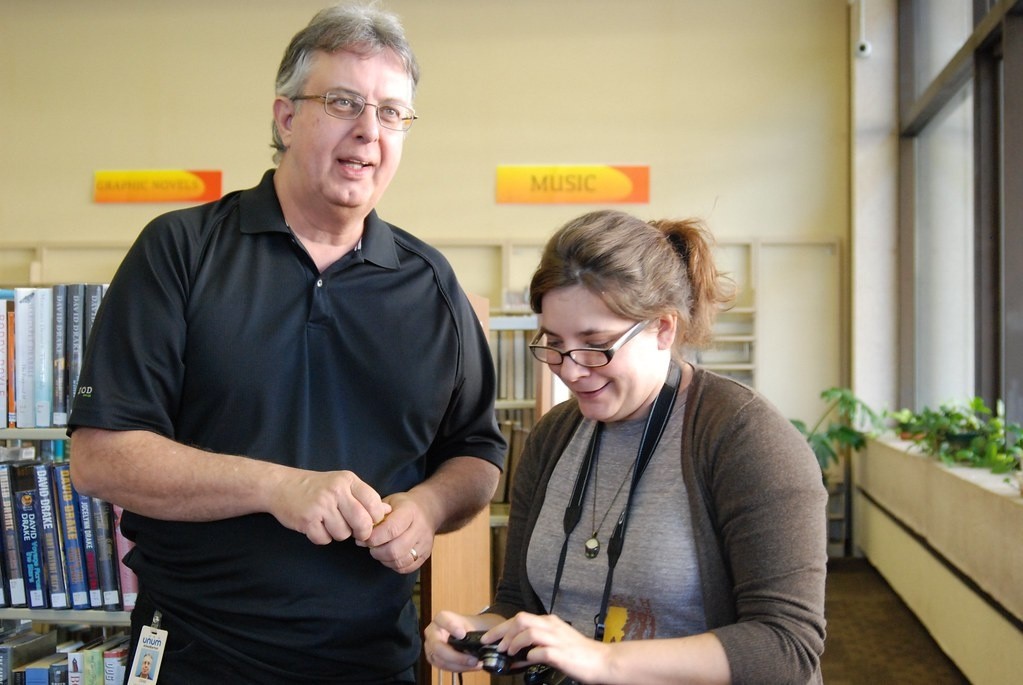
528;313;658;369
287;88;418;132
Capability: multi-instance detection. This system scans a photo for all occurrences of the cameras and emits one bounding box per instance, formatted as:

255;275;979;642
448;632;535;674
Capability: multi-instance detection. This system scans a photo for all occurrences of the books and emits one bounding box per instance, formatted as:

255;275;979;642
489;314;533;401
491;408;532;507
490;526;508;602
0;283;110;429
0;620;133;685
0;438;138;611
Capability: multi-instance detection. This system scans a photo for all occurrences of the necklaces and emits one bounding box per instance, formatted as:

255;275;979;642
583;424;638;559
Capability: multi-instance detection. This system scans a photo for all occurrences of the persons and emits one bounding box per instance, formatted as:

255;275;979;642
424;208;830;685
65;0;508;685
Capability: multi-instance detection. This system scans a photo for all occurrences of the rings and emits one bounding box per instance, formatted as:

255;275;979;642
409;548;419;561
430;650;435;665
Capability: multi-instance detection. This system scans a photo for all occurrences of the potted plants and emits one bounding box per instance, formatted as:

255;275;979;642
922;397;1005;464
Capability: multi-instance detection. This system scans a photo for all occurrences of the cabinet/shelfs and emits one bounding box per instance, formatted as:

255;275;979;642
484;306;757;527
2;428;142;625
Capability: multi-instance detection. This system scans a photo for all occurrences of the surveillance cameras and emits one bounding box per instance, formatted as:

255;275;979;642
858;41;870;56
